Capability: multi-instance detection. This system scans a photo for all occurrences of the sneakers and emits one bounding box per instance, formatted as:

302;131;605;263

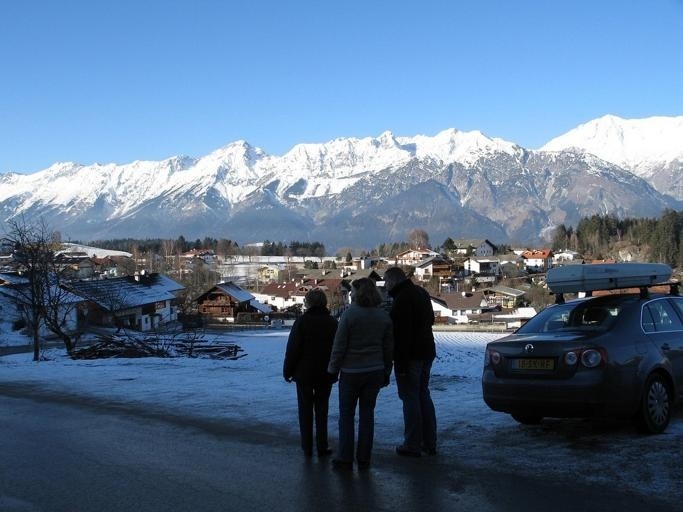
317;449;333;460
331;458;352;471
304;449;313;458
357;457;370;469
396;445;436;458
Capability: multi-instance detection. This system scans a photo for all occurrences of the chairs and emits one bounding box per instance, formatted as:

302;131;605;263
643;306;661;330
584;306;613;325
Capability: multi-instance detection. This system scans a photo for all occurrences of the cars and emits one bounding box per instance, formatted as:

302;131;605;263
479;263;682;434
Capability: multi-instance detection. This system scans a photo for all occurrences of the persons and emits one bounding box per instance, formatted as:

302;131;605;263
327;277;394;468
283;288;339;454
382;267;437;458
